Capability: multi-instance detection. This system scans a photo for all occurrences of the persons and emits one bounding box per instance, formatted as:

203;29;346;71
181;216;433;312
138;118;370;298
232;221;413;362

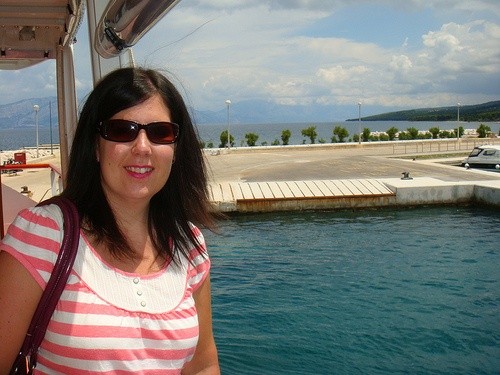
0;67;221;375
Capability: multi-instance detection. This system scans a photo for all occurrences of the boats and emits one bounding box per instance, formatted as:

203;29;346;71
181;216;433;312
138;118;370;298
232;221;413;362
458;144;500;173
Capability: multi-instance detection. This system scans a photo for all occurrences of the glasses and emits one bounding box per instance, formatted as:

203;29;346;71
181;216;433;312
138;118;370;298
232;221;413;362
99;119;180;144
